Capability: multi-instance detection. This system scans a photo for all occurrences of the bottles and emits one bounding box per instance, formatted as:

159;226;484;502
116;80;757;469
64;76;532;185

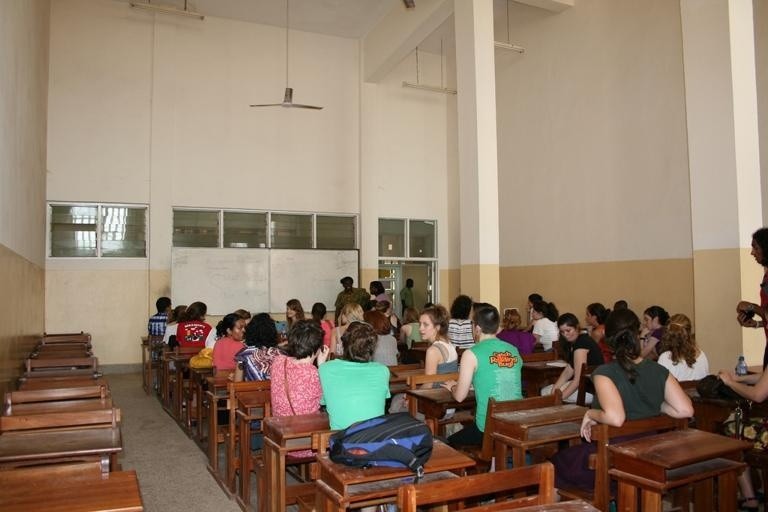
736;356;746;375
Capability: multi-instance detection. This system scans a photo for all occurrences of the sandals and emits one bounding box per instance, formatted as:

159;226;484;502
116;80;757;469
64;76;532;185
740;497;759;511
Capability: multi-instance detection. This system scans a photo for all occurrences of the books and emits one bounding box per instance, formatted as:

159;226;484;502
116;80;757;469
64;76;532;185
543;359;568;367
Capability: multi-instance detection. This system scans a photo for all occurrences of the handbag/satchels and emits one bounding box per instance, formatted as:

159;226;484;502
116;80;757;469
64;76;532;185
696;375;752;409
190;349;213;369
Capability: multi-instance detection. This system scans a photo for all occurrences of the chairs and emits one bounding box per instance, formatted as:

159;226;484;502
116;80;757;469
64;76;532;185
0;332;145;512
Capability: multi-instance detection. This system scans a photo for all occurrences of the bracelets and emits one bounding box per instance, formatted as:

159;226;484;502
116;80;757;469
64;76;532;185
754;320;760;329
451;385;457;392
746;303;757;314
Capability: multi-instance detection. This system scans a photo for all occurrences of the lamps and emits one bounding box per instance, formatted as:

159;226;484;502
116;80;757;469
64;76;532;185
494;39;525;54
129;2;205;21
401;80;458;98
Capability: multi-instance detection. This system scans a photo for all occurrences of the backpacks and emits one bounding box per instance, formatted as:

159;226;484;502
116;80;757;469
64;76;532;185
329;412;432;477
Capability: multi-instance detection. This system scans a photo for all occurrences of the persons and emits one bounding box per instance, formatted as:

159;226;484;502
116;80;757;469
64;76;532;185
439;307;523;452
148;297;329;458
446;293;709;382
716;331;768;511
318;321;391;432
271;277;424;369
526;309;694;493
736;228;767;340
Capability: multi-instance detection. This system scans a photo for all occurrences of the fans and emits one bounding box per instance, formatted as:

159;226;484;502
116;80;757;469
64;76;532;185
248;88;324;110
